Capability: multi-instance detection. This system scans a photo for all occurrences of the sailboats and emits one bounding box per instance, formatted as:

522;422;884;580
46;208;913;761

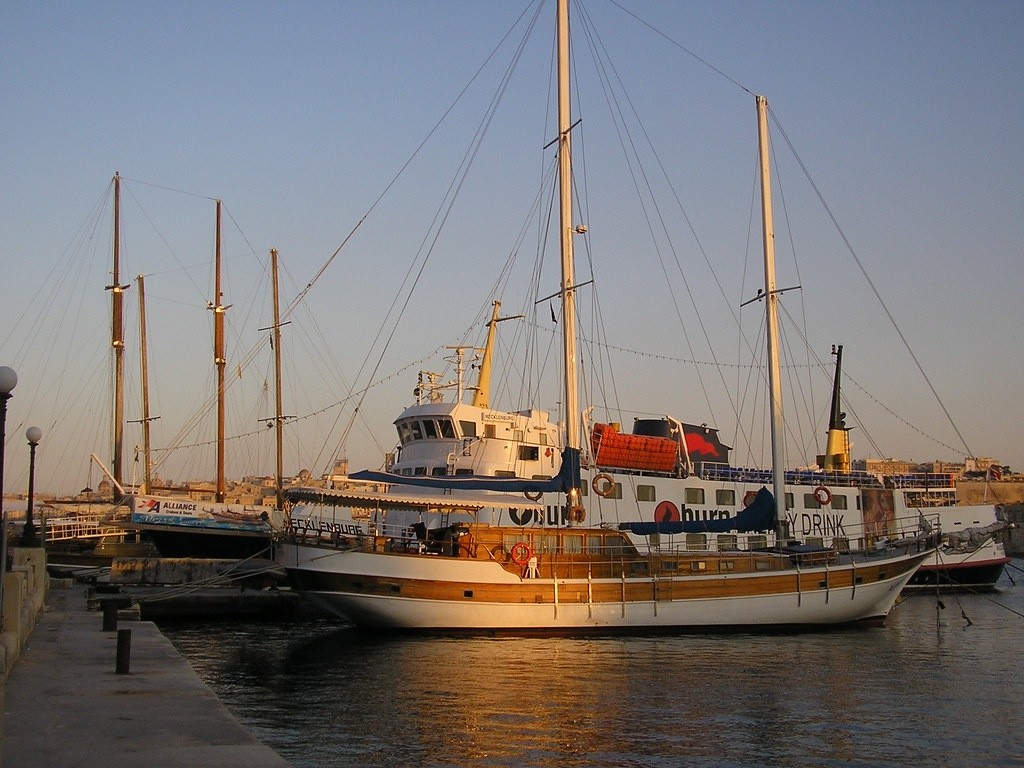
75;1;1007;635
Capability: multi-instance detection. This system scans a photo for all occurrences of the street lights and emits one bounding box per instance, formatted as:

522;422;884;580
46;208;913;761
1;365;19;555
20;427;44;547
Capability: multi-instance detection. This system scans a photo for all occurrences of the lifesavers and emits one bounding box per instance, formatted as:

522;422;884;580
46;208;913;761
815;486;832;505
511;542;531;565
743;492;757;507
592;474;615;497
523;485;543;501
570;506;586;523
